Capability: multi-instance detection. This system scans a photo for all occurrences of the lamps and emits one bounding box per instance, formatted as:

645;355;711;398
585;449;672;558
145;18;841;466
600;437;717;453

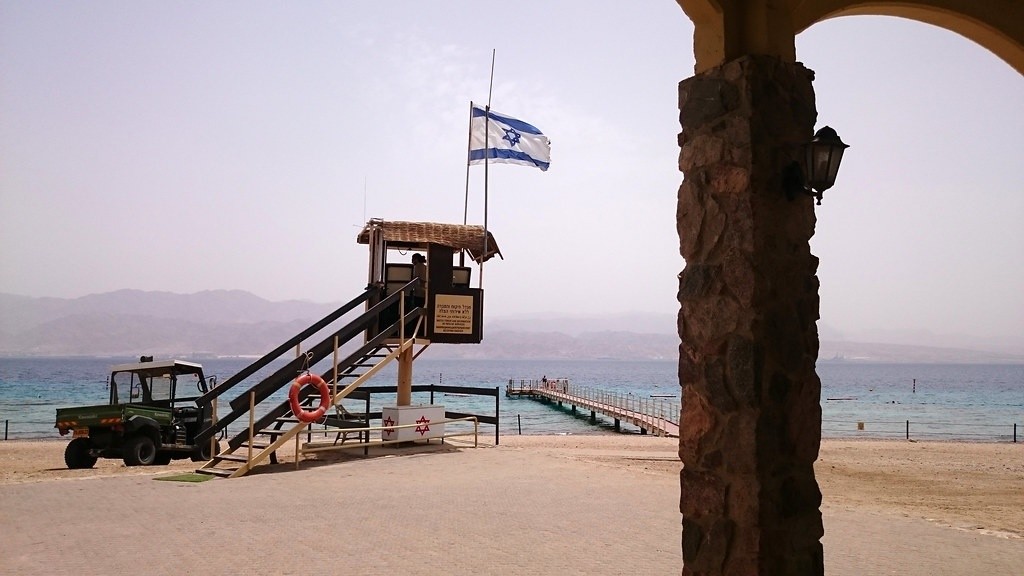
784;126;850;205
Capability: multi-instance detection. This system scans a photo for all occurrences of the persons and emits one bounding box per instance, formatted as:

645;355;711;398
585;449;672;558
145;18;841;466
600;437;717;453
539;375;547;388
411;252;427;306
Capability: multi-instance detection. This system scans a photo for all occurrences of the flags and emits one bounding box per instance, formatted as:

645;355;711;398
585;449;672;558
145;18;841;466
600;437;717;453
469;103;551;172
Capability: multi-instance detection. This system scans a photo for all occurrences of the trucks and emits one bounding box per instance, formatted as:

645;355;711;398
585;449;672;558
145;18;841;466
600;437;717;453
54;356;222;470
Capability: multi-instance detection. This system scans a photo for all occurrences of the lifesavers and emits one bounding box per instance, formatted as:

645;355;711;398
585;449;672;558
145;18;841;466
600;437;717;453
287;373;331;423
